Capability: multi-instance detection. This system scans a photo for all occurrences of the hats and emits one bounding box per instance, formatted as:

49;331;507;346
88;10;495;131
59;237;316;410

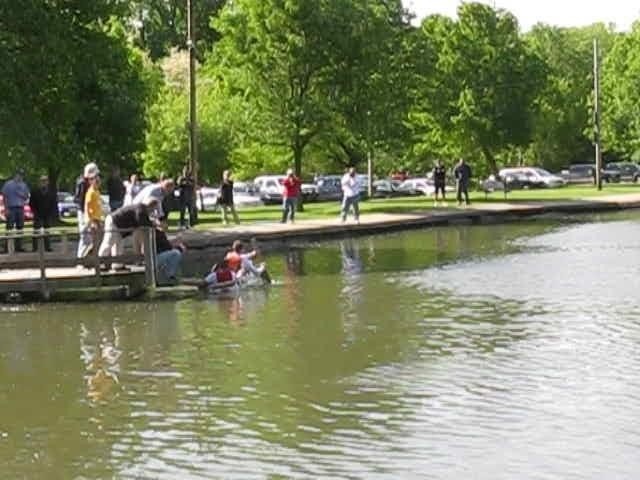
83;161;100;179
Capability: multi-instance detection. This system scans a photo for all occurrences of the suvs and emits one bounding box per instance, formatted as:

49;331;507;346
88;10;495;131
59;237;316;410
606;162;640;183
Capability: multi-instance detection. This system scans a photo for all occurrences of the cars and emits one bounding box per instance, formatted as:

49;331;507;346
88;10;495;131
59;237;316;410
56;191;81;217
197;186;229;214
561;163;606;186
234;172;453;208
483;166;565;190
0;193;34;223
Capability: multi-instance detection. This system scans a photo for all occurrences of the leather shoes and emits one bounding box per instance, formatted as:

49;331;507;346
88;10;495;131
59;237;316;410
114;267;130;272
99;266;108;272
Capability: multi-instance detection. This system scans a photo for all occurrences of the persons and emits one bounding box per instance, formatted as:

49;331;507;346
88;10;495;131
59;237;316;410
206;239;265;283
342;167;360;224
1;166;29;253
219;171;241;225
73;162;187;282
432;159;446;202
177;165;198;226
280;172;299;223
453;158;473;205
29;175;57;252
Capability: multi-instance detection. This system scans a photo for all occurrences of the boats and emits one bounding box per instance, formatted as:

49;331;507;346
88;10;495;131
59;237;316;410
205;236;270;303
